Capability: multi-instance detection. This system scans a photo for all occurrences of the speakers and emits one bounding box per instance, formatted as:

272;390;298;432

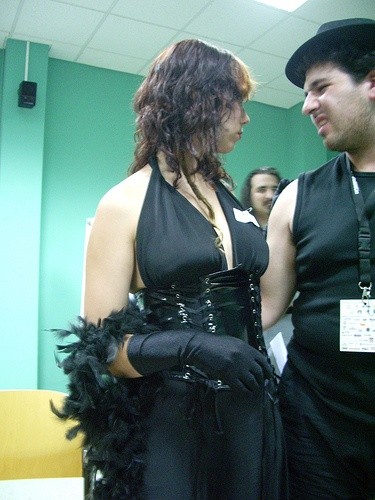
18;80;36;108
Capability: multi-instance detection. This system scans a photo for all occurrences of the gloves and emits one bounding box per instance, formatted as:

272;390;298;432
127;331;272;396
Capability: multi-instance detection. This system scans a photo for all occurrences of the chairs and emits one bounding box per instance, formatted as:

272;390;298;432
0;389;93;479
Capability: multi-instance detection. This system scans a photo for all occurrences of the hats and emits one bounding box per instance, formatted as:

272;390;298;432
285;18;374;88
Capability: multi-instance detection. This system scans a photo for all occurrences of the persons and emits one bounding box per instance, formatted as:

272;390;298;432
79;36;290;500
240;165;296;375
256;13;375;500
